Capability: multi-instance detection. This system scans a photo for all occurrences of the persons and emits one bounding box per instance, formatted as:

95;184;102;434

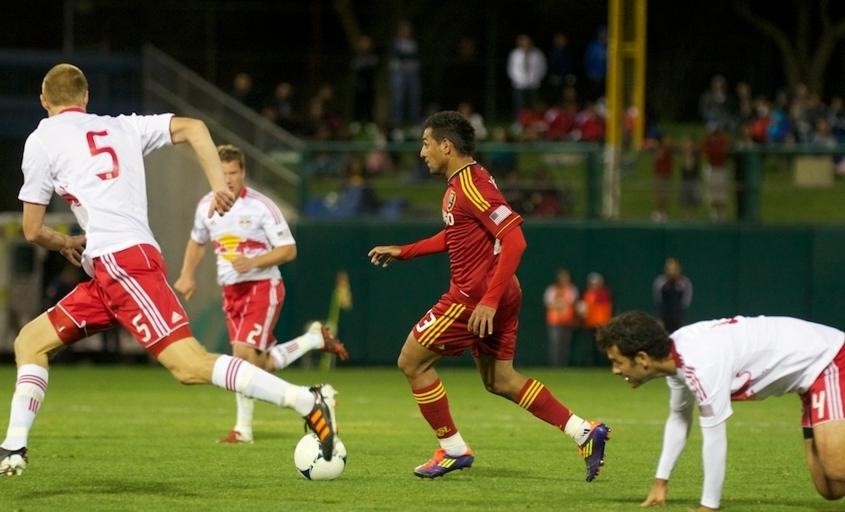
170;143;349;448
352;17;493;139
644;122;758;223
0;63;336;476
706;75;844;146
228;71;344;140
541;270;580;369
496;28;632;143
579;272;613;369
594;313;845;512
653;256;694;335
368;112;613;484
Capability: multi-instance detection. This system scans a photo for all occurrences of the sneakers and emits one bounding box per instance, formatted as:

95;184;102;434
0;443;28;478
579;420;610;482
306;319;351;363
210;431;255;445
301;382;339;460
413;446;477;479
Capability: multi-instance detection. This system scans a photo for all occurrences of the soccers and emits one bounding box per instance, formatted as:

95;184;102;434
294;432;347;480
